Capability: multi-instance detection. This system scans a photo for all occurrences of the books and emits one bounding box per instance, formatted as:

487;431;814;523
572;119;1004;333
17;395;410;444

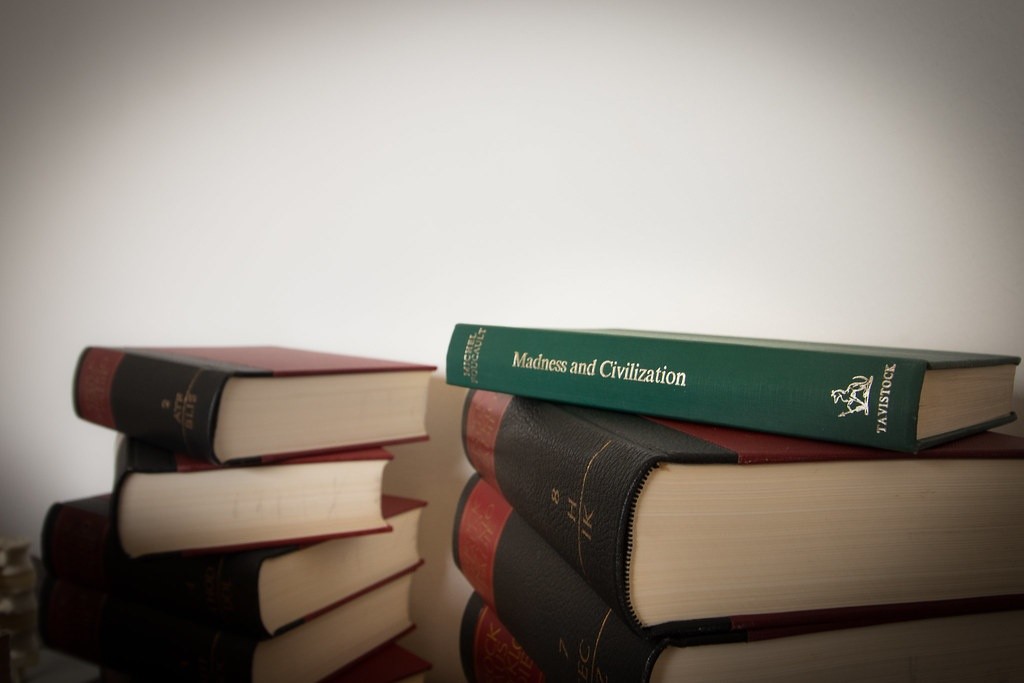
35;348;439;683
442;322;1024;682
